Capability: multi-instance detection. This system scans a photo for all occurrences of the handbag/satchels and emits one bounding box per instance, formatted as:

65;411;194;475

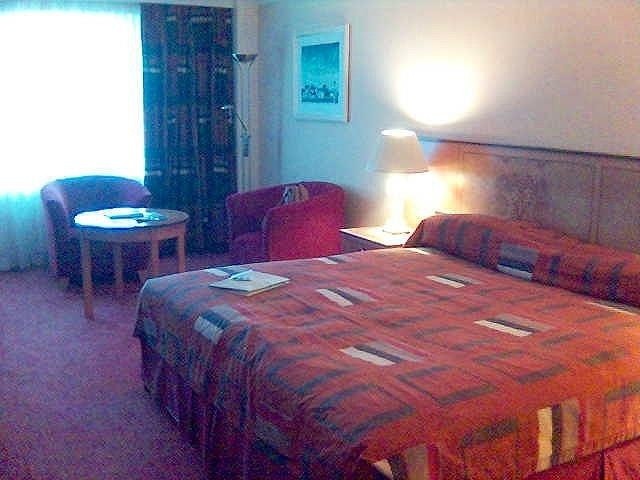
277;184;309;204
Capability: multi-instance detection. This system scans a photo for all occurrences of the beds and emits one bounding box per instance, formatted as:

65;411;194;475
130;242;639;480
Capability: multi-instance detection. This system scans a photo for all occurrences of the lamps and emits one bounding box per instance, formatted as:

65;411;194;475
364;128;432;237
220;52;261;190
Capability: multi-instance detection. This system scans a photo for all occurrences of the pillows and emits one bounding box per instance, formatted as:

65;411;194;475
409;213;638;305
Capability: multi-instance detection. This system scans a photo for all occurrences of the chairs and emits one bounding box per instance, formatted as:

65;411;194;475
225;181;346;262
41;175;152;287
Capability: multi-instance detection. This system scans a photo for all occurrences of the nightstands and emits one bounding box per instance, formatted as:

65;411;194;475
338;220;417;255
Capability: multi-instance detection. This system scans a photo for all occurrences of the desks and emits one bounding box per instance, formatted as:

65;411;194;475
75;209;193;322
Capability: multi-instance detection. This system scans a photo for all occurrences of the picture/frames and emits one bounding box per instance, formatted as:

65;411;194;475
290;22;351;122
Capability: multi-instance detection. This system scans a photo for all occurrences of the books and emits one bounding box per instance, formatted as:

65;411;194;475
209;269;291;298
